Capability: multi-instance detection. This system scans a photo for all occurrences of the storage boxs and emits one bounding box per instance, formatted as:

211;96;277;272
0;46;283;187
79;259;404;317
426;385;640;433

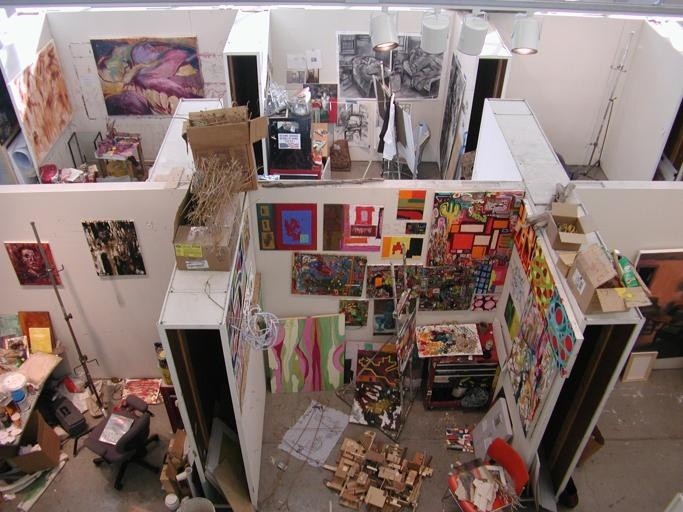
544;198;653;316
0;409;61;475
172;106;269;272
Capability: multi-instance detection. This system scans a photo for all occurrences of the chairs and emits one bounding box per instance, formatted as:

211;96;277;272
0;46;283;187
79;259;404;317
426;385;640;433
84;395;162;491
441;438;529;512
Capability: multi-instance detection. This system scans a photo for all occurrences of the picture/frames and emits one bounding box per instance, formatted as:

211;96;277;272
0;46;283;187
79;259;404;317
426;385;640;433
621;351;658;383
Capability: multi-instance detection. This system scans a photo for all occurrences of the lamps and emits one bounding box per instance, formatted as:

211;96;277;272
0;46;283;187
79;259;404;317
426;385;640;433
367;0;542;58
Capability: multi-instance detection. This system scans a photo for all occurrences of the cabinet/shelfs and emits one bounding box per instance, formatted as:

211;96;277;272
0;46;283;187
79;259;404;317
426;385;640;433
422;323;501;411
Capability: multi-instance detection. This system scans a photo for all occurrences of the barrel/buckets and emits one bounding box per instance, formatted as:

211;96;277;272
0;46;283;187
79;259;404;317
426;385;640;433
4;374;29;405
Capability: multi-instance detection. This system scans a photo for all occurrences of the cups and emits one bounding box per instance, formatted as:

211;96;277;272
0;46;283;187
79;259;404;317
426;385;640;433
11;412;22;426
478;319;490;333
485;339;493;351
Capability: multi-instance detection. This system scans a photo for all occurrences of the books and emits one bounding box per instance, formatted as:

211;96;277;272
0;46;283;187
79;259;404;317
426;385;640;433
0;311;56;367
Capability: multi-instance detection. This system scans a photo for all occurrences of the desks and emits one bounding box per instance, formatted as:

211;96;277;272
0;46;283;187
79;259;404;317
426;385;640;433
1;350;63;445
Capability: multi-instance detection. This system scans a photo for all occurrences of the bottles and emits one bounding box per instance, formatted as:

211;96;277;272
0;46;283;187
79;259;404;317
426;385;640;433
164;495;180;510
79;166;90;183
153;341;167;367
292;93;307;117
262;89;276;115
95;134;114;157
0;410;10;435
